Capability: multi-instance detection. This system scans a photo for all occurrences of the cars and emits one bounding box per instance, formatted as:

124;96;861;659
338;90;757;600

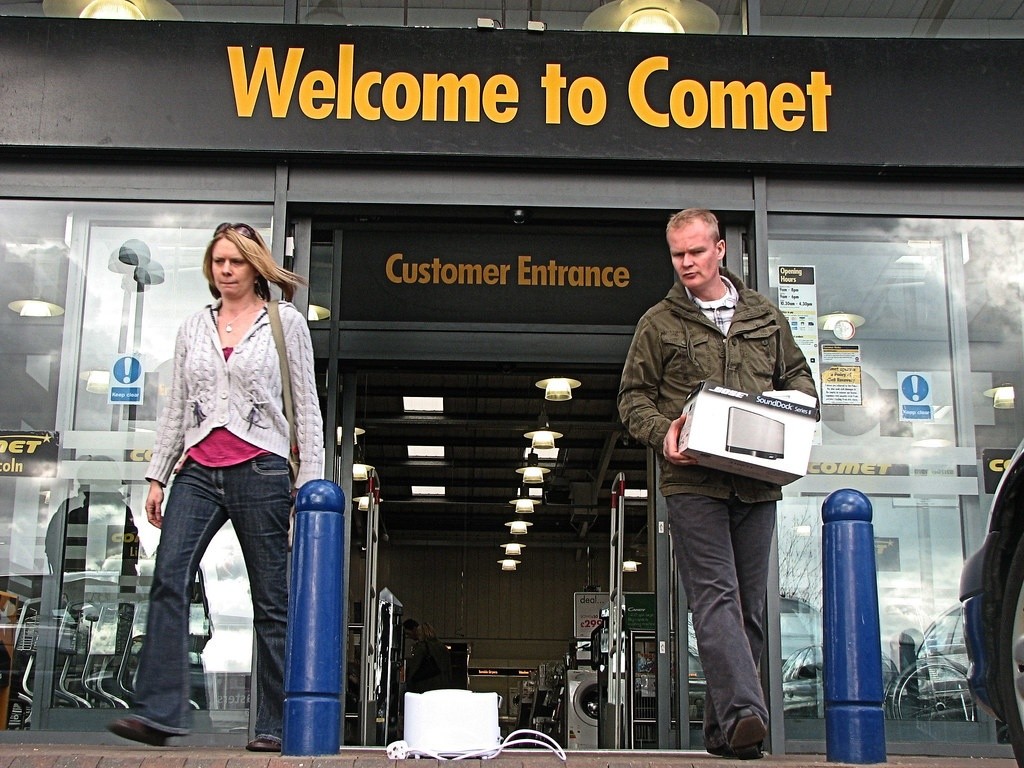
916;606;969;705
687;593;824;719
961;440;1024;767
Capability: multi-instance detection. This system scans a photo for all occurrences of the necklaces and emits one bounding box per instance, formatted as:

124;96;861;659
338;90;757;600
221;302;256;332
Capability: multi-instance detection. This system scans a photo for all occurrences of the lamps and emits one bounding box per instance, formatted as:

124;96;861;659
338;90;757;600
504;515;533;535
508;484;541;514
9;265;66;318
983;382;1015;409
516;448;551;484
308;304;330;321
523;404;563;449
346;445;375;481
353;483;384;511
623;554;643;572
500;535;527;555
497;556;521;571
817;311;866;331
535;377;582;401
336;423;366;446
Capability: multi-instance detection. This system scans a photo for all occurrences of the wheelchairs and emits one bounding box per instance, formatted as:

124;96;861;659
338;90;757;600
883;653;976;722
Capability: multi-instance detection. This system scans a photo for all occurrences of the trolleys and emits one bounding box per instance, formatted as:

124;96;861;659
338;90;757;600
10;593;211;729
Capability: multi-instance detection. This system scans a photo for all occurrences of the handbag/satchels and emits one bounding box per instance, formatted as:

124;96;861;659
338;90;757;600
411;640;441;684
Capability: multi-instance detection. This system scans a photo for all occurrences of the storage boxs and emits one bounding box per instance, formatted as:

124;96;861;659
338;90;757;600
677;380;821;486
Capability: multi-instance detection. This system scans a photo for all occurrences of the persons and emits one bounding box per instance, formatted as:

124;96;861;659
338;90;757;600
103;223;324;753
403;619;442;691
616;205;821;760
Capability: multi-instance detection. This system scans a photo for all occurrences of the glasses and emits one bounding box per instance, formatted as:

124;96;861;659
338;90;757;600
212;222;261;246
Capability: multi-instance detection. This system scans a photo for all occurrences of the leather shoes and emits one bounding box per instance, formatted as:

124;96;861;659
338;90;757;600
727;708;765;760
245;737;280;753
105;718;166;745
